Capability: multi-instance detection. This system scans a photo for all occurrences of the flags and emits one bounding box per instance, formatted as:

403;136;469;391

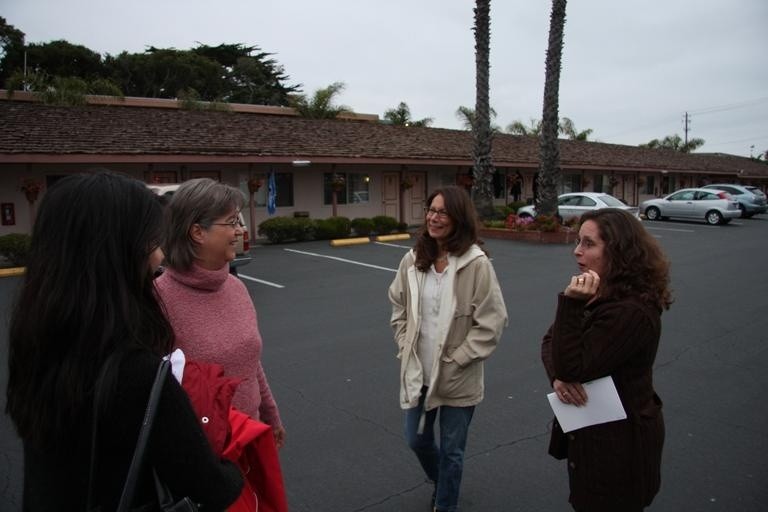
266;168;278;217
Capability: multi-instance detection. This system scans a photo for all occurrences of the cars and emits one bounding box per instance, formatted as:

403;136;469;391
516;187;641;228
639;188;744;225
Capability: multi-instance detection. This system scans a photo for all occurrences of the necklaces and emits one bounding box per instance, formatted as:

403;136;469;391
435;254;448;264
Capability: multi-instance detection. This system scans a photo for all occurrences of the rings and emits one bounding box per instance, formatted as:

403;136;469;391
577;278;585;284
562;392;568;396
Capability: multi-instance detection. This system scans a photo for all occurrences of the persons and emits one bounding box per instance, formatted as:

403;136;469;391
5;171;247;512
540;207;676;512
464;175;473;198
494;183;504;199
388;185;510;512
510;171;539;203
151;177;287;512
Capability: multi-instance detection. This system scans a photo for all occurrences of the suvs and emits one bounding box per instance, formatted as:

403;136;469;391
682;183;767;219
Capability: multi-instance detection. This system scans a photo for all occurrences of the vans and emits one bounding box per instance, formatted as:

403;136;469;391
145;183;253;280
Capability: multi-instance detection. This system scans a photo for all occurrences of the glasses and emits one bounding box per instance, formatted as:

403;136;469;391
208;219;240;227
573;237;605;250
424;208;449;217
